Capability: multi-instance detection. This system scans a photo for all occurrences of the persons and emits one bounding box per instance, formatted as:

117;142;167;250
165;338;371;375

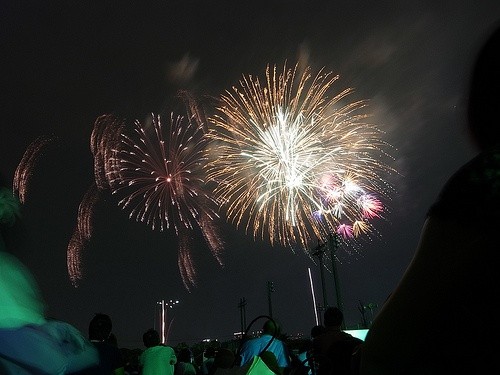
359;27;500;375
1;251;365;375
